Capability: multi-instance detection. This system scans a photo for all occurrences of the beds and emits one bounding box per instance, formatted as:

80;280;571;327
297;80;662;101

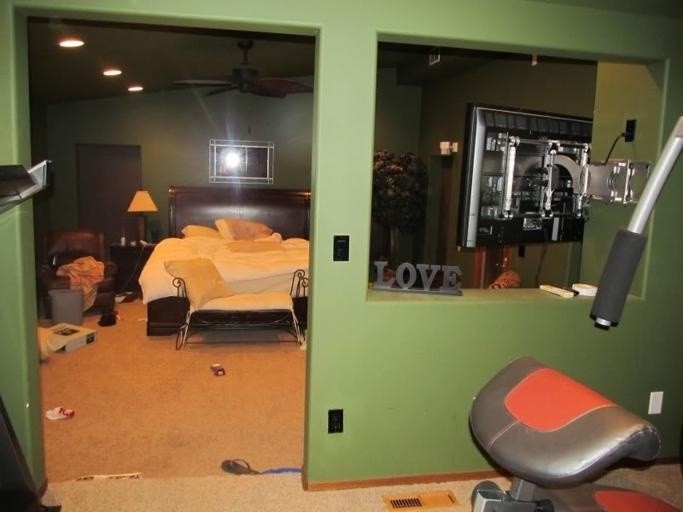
147;186;310;337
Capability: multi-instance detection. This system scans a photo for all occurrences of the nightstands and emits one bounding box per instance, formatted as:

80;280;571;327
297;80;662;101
108;242;157;294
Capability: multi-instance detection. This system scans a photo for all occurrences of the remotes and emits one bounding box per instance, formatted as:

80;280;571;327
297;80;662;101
539;283;579;298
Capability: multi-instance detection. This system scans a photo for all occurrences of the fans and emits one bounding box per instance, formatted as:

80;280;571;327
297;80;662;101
171;39;312;103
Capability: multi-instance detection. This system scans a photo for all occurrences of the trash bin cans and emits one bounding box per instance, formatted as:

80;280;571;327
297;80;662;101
48;288;83;326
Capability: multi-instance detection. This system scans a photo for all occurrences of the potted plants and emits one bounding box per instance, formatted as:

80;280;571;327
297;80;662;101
373;148;430;289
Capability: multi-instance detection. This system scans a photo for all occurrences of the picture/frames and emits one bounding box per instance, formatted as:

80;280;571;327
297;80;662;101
207;138;274;186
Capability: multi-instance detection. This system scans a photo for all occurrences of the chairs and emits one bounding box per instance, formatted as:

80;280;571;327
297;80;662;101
36;228;118;319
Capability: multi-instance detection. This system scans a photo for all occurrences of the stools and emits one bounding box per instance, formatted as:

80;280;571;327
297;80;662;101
175;269;309;347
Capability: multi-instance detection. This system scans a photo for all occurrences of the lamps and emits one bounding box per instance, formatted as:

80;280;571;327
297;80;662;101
125;190;158;241
439;137;460;157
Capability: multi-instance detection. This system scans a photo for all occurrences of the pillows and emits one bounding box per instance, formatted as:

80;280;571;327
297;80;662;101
162;258;236;310
181;218;275;242
